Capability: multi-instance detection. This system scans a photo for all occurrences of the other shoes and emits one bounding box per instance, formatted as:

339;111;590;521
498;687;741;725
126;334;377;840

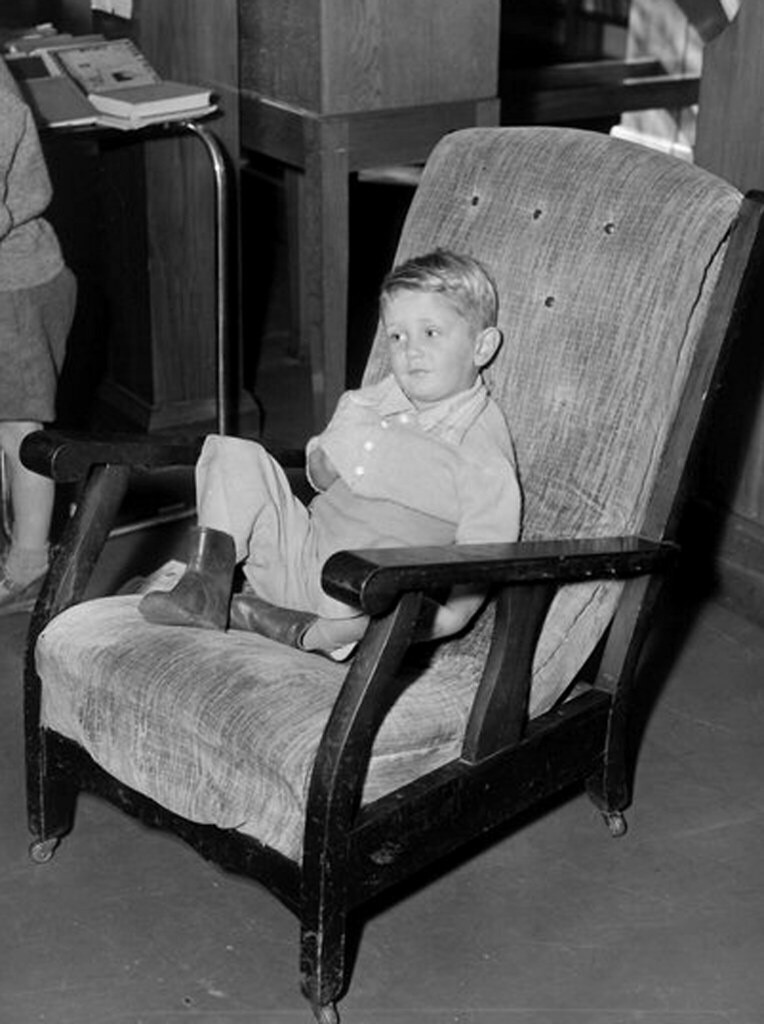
0;558;50;618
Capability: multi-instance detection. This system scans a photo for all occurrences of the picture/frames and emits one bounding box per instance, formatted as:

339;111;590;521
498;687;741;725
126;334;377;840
39;40;162;97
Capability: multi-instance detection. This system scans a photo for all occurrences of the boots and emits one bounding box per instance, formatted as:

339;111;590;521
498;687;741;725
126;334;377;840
225;591;318;655
137;523;233;632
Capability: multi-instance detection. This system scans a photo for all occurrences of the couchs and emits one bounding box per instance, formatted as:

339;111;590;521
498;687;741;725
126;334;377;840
32;125;764;1009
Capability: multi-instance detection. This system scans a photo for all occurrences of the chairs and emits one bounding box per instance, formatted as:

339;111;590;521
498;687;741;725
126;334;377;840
11;119;241;838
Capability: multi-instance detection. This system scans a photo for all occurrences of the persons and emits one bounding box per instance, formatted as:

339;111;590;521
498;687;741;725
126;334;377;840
1;58;78;615
139;250;522;661
